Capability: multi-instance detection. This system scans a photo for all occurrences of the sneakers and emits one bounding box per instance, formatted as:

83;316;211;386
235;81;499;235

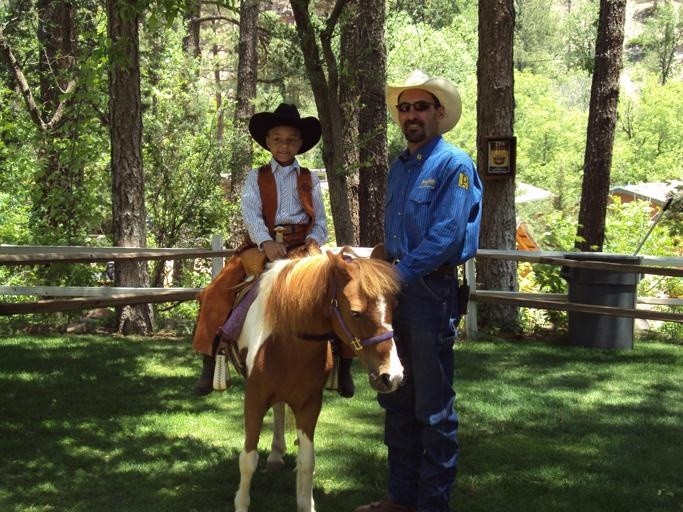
355;496;416;512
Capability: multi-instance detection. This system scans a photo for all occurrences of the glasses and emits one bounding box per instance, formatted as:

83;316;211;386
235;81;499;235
395;100;438;112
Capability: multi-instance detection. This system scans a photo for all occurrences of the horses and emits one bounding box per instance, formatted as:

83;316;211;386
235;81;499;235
220;242;406;512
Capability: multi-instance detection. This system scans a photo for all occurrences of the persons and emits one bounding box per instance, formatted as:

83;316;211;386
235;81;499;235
358;89;483;510
193;119;355;399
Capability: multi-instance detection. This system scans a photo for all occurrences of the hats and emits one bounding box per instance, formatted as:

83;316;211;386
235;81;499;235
384;69;461;134
249;103;321;154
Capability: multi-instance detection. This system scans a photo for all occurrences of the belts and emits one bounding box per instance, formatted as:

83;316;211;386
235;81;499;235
284;225;305;234
388;257;457;273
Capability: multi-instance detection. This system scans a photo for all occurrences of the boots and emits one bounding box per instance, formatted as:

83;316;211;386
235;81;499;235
337;357;355;398
193;354;215;396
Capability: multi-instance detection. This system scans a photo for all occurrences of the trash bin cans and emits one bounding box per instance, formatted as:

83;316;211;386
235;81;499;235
561;253;643;349
